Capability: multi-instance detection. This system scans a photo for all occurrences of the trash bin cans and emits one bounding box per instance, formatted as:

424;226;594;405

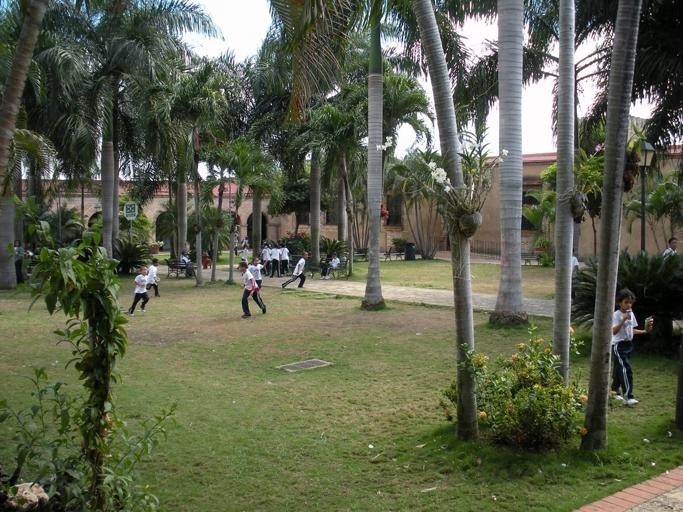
405;243;416;261
677;345;683;430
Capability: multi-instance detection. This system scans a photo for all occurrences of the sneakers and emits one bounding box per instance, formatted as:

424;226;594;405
263;271;289;278
140;304;145;312
128;308;134;317
262;304;266;314
155;293;161;297
319;276;330;280
240;313;253;318
612;392;638;406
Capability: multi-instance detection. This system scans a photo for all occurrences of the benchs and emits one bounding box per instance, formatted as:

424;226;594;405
164;258;198;279
286;254;347;281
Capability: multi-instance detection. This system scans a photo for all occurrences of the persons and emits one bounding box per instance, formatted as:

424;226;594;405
320;252;341;280
282;252;308;288
241;236;291;290
610;287;654;407
180;250;193;277
129;264;149;316
572;248;579;300
238;262;266;318
663;238;678;261
146;258;160;297
14;240;24;284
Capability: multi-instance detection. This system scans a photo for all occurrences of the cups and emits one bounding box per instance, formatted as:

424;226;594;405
626;310;632;320
